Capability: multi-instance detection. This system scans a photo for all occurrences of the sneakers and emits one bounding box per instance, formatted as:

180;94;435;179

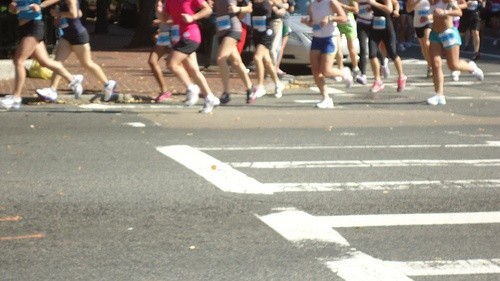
369;81;384;92
469;60;483;82
256;84;266;97
246;85;257;104
219;93;230;105
69;75;83;99
185;85;200;105
342;67;353;88
0;95;21;108
381;57;390;78
453;71;461;81
427;66;434;78
427;95;446;105
198;96;220;113
315;97;334;108
275;83;285;97
36;87;57;101
155;92;172;101
104;80;116;101
356;74;367;85
397;74;407;91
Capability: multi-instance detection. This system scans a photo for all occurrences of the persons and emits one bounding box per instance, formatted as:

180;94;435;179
35;0;116;103
302;0;355;110
335;0;358;89
353;0;390;85
451;0;467;81
361;0;406;95
459;0;500;82
165;0;219;113
148;0;173;103
403;0;434;79
264;0;295;79
0;0;83;106
208;0;256;105
423;0;484;105
248;0;286;99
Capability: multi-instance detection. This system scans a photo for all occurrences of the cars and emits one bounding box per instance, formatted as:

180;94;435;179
280;0;361;66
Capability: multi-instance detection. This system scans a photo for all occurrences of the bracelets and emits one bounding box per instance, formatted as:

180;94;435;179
239;6;242;13
444;9;448;17
328;15;332;22
481;1;483;4
